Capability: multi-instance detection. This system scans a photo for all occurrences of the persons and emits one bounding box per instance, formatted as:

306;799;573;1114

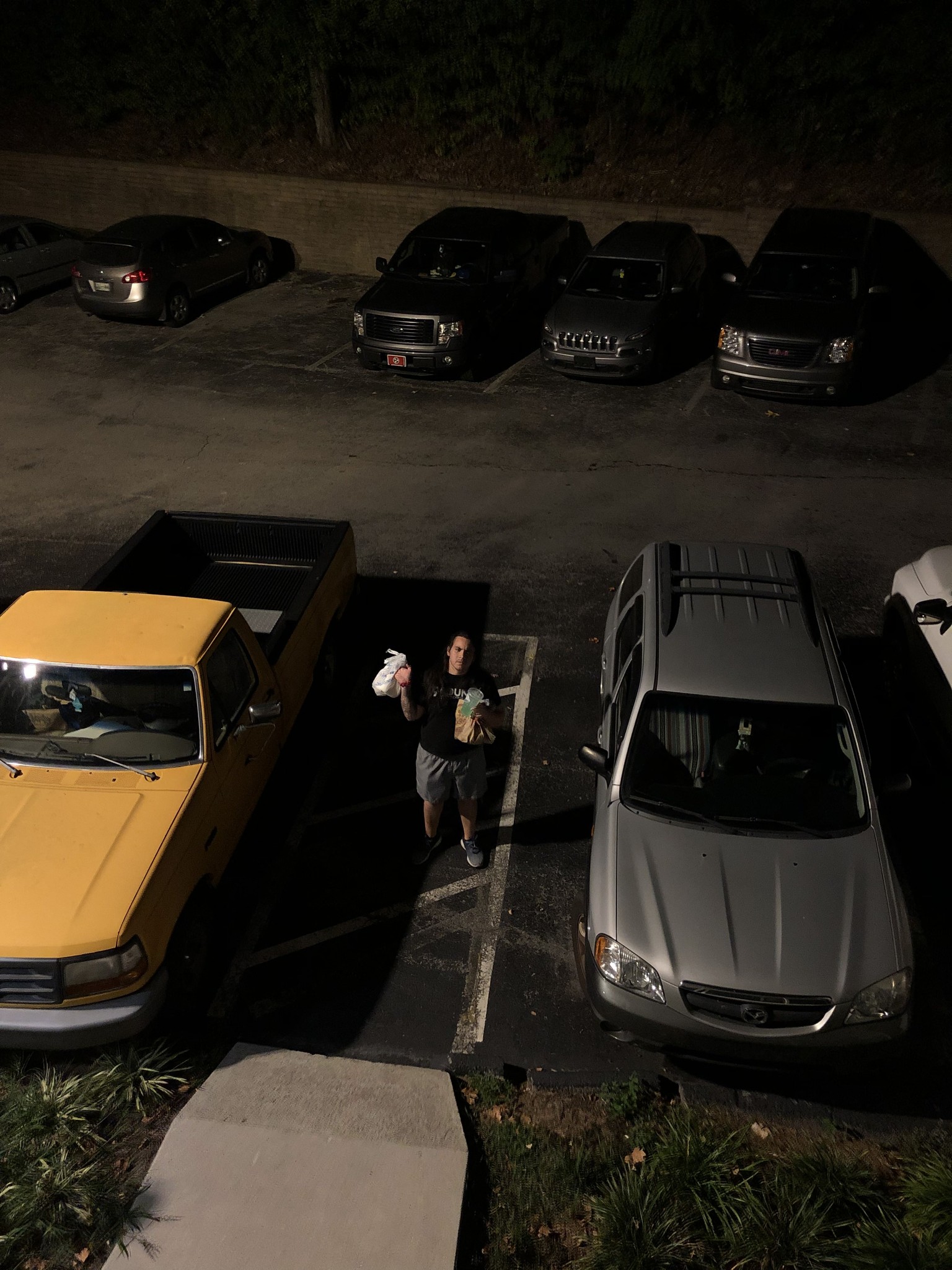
394;635;505;870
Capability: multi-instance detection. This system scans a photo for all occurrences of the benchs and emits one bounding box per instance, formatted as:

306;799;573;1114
78;681;154;712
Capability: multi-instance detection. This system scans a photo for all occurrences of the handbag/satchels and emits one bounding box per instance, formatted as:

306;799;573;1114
372;649;407;698
454;699;496;745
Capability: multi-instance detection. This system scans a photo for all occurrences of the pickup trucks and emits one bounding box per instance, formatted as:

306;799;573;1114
0;508;358;1051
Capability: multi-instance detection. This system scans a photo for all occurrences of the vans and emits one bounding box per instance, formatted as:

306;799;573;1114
572;539;914;1056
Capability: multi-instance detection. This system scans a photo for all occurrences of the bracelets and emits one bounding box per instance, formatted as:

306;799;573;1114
399;680;411;688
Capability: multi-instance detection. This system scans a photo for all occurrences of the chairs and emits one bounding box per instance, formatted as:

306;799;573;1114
154;687;191;711
473;250;488;267
643;706;711;789
602;259;664;299
434;246;454;269
11;234;27;251
206;651;244;695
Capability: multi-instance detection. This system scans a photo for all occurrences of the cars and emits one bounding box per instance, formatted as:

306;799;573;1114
710;209;879;397
70;214;272;327
0;214;98;314
351;207;535;374
882;546;952;701
540;221;701;377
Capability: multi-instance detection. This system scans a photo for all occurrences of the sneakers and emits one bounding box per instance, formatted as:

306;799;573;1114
460;835;483;867
413;834;442;864
